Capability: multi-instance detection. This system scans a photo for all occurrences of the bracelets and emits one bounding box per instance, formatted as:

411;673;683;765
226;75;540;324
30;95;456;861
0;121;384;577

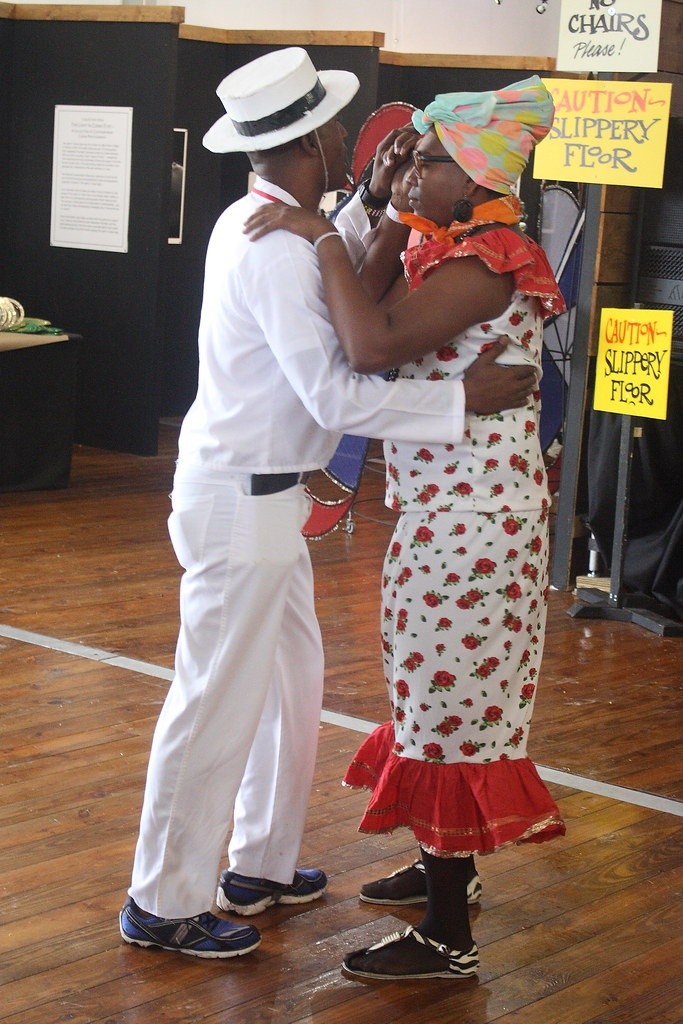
361;203;385;218
314;231;342;255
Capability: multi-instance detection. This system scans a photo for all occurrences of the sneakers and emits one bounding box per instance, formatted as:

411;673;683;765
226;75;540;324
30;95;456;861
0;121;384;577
216;869;328;916
118;895;262;958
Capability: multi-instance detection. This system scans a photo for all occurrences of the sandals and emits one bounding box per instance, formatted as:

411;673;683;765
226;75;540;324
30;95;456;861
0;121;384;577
342;924;480;980
360;863;483;903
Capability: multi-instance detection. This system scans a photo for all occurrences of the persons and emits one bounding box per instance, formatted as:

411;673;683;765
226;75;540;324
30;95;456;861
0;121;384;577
244;74;566;983
118;45;537;959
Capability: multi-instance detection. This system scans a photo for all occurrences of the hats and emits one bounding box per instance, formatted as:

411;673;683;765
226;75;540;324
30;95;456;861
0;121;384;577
202;46;360;154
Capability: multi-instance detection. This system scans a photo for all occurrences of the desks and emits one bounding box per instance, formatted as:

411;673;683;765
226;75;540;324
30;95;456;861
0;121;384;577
0;324;73;494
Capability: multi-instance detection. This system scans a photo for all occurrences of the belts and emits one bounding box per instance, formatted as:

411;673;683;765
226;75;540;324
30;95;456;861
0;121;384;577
249;472;304;495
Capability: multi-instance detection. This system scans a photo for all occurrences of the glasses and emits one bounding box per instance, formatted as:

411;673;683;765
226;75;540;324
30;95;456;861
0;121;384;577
412;150;455;179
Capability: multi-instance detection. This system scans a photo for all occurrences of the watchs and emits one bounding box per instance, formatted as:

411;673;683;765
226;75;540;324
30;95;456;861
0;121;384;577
359;179;394;210
